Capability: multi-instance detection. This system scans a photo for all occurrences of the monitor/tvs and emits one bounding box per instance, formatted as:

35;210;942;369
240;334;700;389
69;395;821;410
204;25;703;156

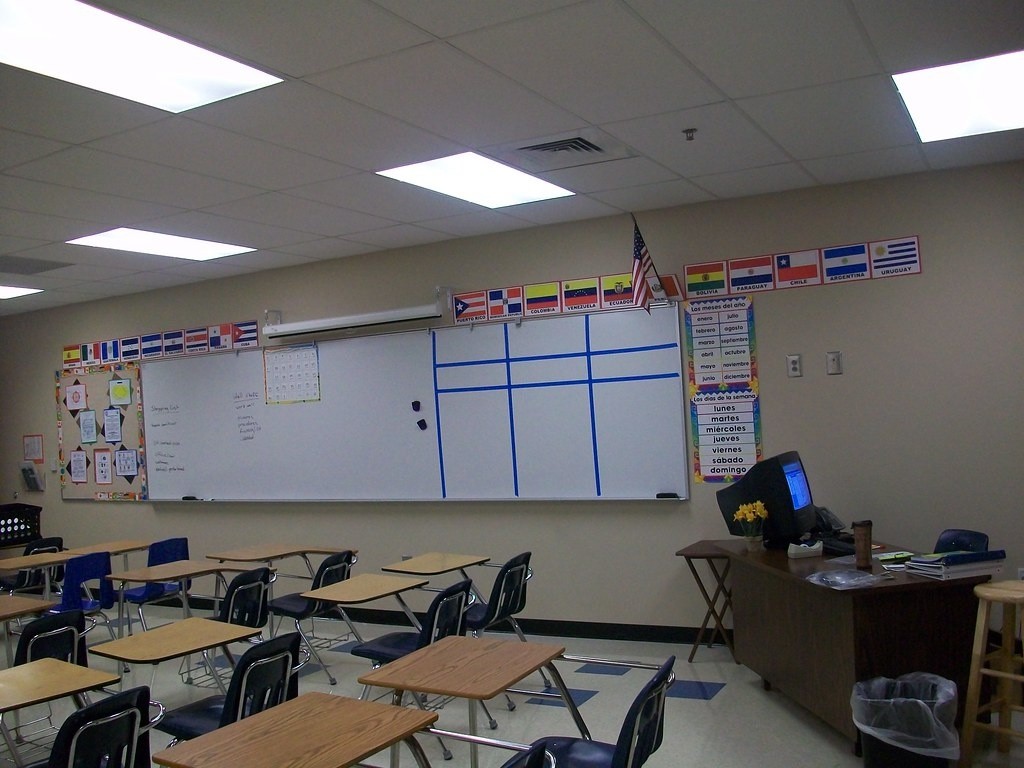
715;450;817;550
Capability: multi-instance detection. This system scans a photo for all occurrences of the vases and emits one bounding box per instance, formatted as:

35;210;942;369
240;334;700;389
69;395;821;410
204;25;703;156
744;535;765;553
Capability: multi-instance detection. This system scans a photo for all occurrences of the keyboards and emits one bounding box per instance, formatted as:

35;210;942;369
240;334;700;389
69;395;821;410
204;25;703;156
826;538;858;556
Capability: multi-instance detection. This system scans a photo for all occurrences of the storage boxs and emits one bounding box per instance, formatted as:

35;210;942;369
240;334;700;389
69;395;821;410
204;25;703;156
0;502;44;549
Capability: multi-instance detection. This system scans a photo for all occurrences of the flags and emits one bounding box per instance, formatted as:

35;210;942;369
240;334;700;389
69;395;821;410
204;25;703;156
631;222;653;317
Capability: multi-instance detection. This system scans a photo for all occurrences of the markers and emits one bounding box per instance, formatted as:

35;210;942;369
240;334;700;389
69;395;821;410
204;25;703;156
200;498;215;501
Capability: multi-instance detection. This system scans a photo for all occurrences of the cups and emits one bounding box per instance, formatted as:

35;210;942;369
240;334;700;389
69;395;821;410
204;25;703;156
850;520;873;571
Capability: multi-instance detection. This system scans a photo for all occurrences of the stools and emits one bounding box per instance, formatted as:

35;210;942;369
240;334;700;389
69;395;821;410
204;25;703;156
958;579;1024;768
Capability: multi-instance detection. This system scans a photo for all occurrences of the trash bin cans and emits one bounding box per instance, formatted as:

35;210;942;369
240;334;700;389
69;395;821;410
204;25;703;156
852;672;960;768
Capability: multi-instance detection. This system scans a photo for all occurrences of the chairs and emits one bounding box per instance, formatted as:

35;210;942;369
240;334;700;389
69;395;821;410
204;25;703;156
0;536;678;768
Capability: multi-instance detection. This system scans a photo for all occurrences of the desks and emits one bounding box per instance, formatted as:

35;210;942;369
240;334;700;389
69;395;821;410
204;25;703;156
0;658;121;768
104;560;278;684
0;594;56;743
65;539;149;637
714;540;991;757
207;543;359;664
381;551;491;605
1;553;86;595
152;691;440;768
677;540;733;662
88;616;262;697
300;573;429;633
356;635;590;768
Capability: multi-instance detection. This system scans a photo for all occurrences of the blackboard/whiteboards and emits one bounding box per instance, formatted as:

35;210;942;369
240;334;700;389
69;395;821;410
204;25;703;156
141;303;689;502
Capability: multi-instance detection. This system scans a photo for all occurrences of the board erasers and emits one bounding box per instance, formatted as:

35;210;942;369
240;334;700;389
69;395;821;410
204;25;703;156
656;492;678;498
182;496;196;500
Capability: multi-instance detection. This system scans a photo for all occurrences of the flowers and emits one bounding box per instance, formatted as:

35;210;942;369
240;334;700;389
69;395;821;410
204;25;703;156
733;501;769;537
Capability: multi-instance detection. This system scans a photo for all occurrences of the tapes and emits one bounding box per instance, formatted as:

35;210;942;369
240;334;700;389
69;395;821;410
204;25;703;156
799;544;810;549
